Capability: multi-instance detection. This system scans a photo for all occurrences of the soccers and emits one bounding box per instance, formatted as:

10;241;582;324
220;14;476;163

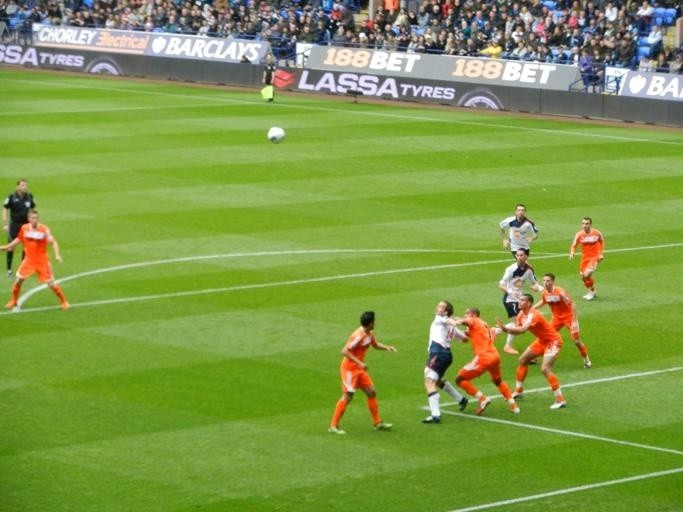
268;127;285;144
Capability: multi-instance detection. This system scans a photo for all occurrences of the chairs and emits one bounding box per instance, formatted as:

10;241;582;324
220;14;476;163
0;0;678;72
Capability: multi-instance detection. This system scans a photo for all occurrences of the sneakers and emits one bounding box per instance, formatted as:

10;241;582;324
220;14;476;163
505;386;523;401
372;423;393;431
327;425;347;435
60;300;69;310
550;396;568;410
458;396;469;412
502;344;519;355
6;299;19;309
421;415;441;424
583;290;597;301
506;402;521;415
583;361;593;369
476;395;491;416
6;269;13;278
490;327;497;343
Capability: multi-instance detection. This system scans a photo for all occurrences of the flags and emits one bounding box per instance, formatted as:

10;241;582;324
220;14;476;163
261;85;273;99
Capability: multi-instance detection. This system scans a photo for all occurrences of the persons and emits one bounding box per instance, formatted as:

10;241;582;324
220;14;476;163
499;205;539;263
0;209;69;310
569;217;603;300
491;248;544;353
1;1;683;84
328;312;397;435
263;54;275;102
455;307;520;416
497;294;567;411
422;300;469;424
3;179;36;274
529;273;592;367
